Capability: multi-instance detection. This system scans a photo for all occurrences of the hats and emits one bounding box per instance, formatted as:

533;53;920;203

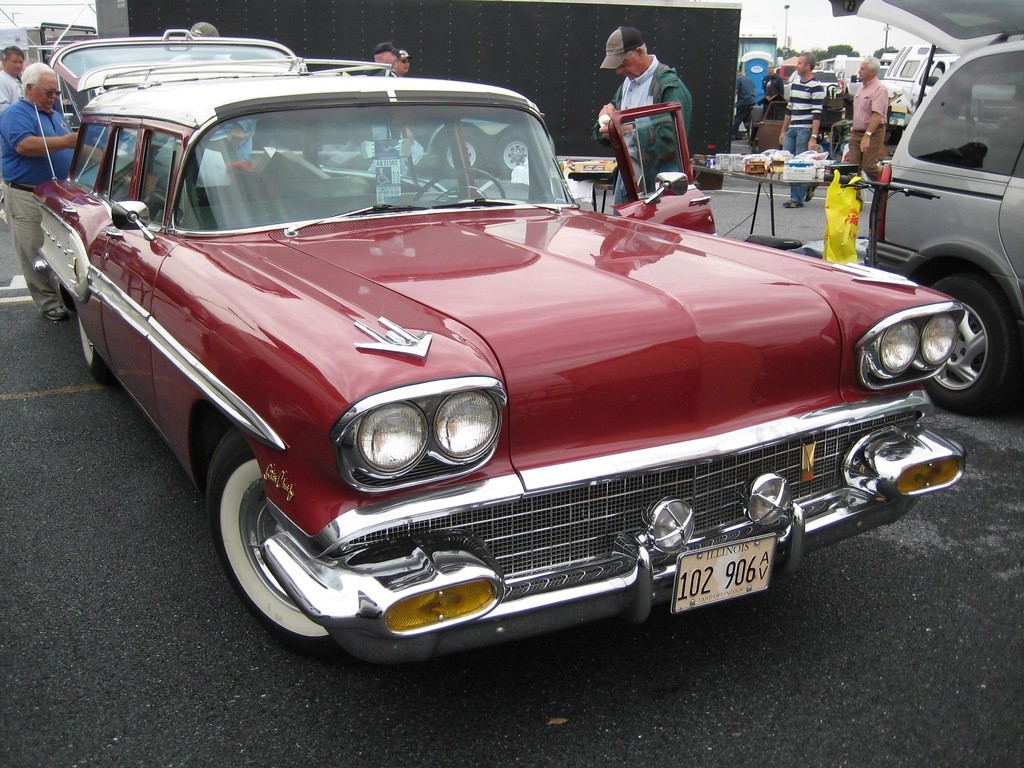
189;22;220;37
600;26;645;69
398;50;411;60
372;41;400;55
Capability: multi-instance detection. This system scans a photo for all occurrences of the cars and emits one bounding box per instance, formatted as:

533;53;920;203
27;28;971;661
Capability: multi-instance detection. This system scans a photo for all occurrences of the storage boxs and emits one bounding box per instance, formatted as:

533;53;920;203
253;140;332;201
886;92;912;126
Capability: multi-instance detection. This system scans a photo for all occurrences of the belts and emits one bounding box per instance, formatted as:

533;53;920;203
3;180;34;193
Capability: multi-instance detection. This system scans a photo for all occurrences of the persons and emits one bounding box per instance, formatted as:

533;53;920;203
850;55;889;211
0;63;79;320
778;53;825;207
592;27;698;216
372;42;413;176
0;46;28;107
763;68;785;102
733;71;757;140
145;22;259;219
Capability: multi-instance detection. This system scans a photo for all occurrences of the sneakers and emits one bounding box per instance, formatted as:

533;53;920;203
44;307;69;321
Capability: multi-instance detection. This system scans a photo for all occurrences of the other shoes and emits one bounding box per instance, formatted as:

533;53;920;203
782;200;802;208
804;186;816;202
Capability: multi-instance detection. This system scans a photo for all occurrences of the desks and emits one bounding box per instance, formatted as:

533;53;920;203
568;164;620;213
691;163;824;245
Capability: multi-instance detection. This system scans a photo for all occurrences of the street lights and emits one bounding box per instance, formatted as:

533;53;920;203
783;3;791;58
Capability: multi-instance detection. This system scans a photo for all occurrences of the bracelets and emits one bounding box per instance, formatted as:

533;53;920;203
811;133;818;138
866;132;872;136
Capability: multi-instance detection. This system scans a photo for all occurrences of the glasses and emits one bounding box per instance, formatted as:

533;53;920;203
620;53;634;68
35;84;61;97
400;59;410;63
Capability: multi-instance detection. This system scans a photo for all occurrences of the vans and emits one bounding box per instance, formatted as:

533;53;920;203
862;37;1024;415
776;43;1017;142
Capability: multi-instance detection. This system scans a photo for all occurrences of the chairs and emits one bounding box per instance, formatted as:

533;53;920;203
323;177;372;218
